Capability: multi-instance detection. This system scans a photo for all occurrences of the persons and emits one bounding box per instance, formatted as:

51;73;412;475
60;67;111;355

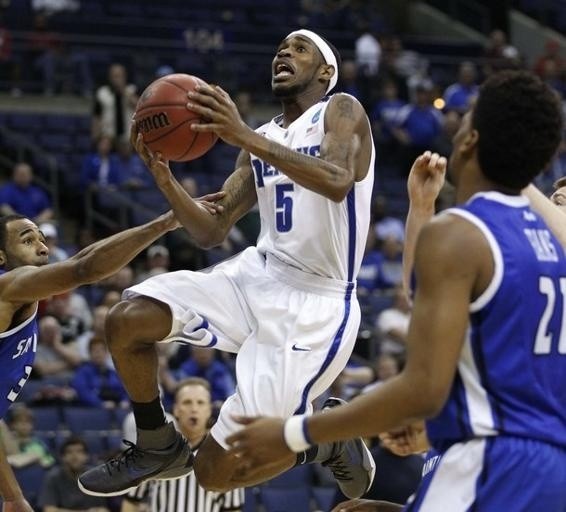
117;376;247;509
75;31;393;500
227;68;565;510
1;188;226;510
30;436;109;509
0;29;565;466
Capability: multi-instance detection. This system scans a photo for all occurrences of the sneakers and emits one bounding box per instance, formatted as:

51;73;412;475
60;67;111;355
321;397;376;499
78;432;195;498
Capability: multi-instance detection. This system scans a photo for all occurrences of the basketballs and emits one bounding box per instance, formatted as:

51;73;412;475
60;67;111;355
137;73;220;162
